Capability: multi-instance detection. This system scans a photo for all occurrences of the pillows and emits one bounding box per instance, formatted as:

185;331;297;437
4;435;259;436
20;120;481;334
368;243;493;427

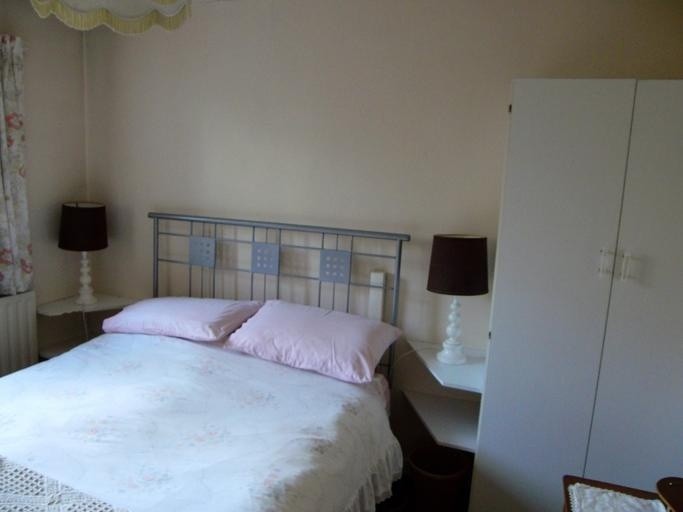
224;299;403;383
103;296;262;341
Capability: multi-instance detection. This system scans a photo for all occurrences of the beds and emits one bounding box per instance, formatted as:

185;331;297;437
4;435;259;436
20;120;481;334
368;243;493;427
0;212;410;512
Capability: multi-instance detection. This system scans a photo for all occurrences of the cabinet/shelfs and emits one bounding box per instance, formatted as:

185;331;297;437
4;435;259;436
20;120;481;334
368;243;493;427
470;78;683;512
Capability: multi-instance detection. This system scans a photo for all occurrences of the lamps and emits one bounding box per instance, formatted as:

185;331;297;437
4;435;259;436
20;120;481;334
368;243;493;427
58;200;109;305
424;232;490;363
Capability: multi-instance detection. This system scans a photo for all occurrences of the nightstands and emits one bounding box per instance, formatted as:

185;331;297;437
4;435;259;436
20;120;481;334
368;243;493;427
39;292;133;357
402;338;482;454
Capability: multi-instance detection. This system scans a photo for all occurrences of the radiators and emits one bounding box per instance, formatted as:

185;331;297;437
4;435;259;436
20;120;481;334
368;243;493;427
0;291;39;377
